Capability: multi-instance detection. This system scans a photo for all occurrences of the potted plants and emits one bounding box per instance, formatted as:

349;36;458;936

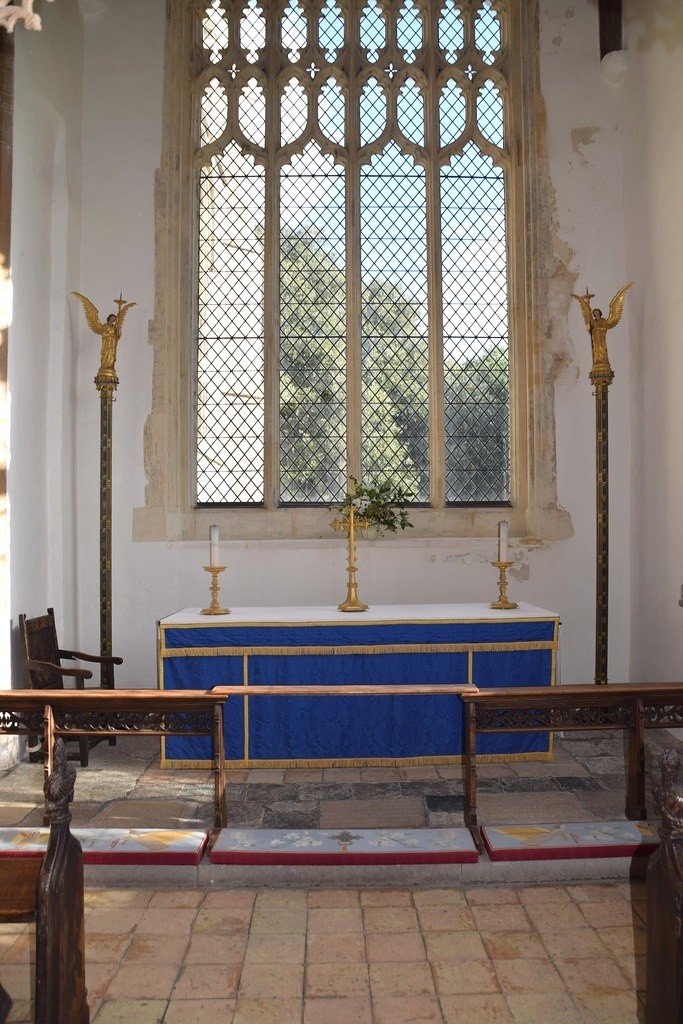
334;476;407;539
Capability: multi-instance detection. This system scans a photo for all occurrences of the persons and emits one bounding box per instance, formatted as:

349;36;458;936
101;314;118;370
587;308;609;365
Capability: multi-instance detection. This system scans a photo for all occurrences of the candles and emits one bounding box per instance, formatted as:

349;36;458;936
210;523;219;568
498;519;508;562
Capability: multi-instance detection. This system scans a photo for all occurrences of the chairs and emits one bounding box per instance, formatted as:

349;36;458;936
19;608;124;764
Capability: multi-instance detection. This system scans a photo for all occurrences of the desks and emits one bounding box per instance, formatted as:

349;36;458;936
157;603;560;761
